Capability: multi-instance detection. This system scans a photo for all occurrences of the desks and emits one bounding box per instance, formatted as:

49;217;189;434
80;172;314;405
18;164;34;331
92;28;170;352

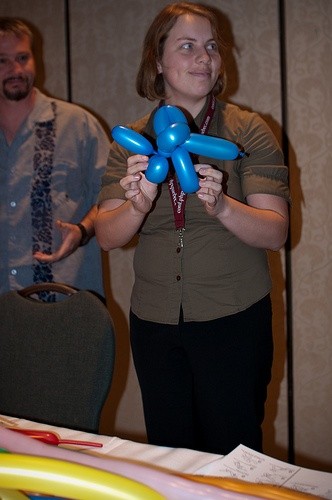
0;414;332;500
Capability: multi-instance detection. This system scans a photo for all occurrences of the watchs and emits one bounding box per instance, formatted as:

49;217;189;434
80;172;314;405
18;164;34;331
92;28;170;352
77;223;90;247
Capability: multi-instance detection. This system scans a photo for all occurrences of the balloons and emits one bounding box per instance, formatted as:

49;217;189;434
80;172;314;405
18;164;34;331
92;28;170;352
112;106;245;193
0;419;328;499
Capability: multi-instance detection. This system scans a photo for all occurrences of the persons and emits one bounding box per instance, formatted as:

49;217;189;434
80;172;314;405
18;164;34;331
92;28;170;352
1;15;112;315
95;1;290;457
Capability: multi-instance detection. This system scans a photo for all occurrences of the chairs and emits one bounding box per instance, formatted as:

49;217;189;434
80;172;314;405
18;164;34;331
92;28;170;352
1;284;130;437
